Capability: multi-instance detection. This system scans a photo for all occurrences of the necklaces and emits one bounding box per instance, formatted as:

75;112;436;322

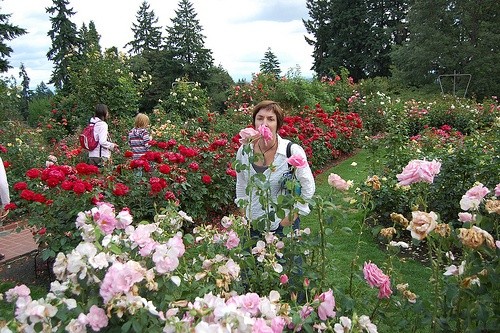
258;138;276;166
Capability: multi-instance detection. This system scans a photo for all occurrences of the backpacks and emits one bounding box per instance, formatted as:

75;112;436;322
79;120;103;151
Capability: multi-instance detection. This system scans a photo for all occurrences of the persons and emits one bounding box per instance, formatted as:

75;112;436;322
236;101;315;304
88;104;120;168
0;156;11;261
127;113;153;182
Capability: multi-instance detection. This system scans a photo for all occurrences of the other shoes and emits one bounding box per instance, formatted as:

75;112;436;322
0;254;5;260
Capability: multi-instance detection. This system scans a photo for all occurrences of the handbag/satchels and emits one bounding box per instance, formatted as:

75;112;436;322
284;142;301;197
